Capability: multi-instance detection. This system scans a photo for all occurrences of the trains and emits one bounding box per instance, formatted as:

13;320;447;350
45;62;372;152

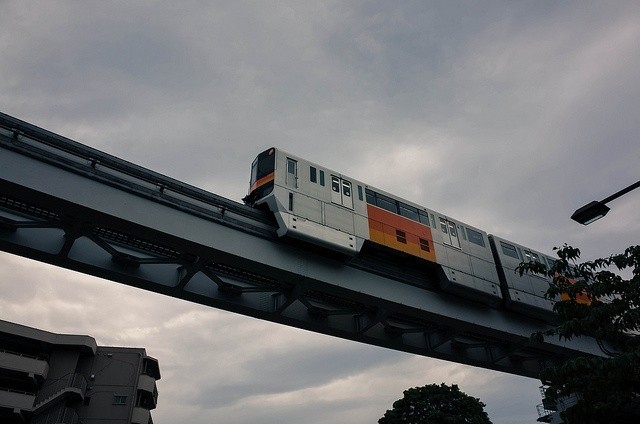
241;146;640;336
